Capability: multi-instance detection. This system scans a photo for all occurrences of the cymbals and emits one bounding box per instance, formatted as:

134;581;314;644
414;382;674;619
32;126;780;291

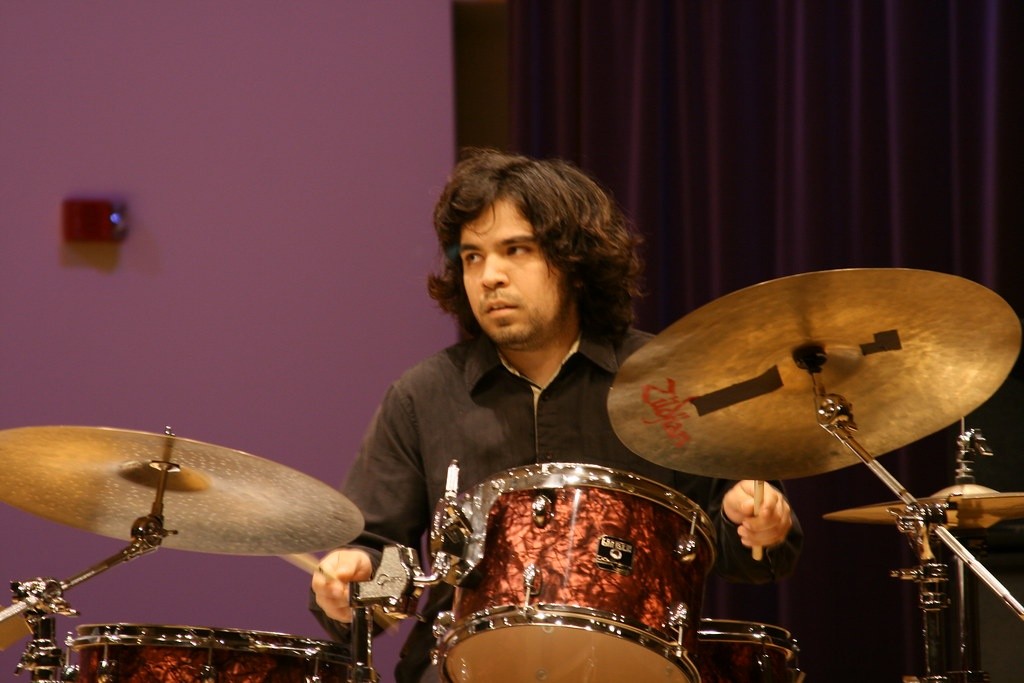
607;268;1022;480
0;425;365;556
822;484;1024;529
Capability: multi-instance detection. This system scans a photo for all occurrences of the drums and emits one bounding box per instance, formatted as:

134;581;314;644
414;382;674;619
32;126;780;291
430;462;719;683
72;623;382;683
694;618;800;683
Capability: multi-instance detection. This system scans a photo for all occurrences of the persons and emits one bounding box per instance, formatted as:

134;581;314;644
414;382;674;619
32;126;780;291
311;146;807;683
